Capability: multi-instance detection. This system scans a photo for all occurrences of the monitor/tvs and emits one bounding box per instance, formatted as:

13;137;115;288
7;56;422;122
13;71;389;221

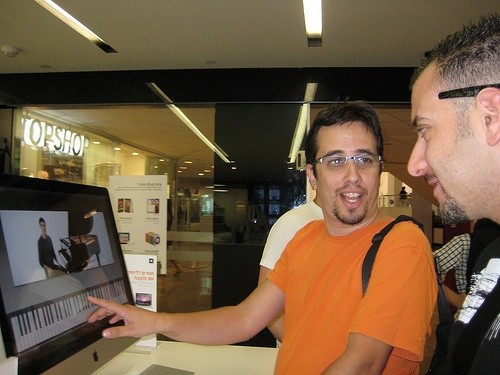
0;173;141;375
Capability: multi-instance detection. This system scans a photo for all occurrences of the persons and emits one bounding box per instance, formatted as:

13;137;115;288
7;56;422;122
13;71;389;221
258;159;325;343
400;187;407;199
36;215;68;280
432;231;473;309
408;16;500;375
86;103;439;375
164;198;182;276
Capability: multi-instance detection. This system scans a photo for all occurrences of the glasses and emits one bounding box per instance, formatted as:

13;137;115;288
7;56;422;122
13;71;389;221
313;154;383;167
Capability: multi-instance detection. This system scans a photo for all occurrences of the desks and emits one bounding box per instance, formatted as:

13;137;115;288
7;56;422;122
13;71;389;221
92;338;280;375
